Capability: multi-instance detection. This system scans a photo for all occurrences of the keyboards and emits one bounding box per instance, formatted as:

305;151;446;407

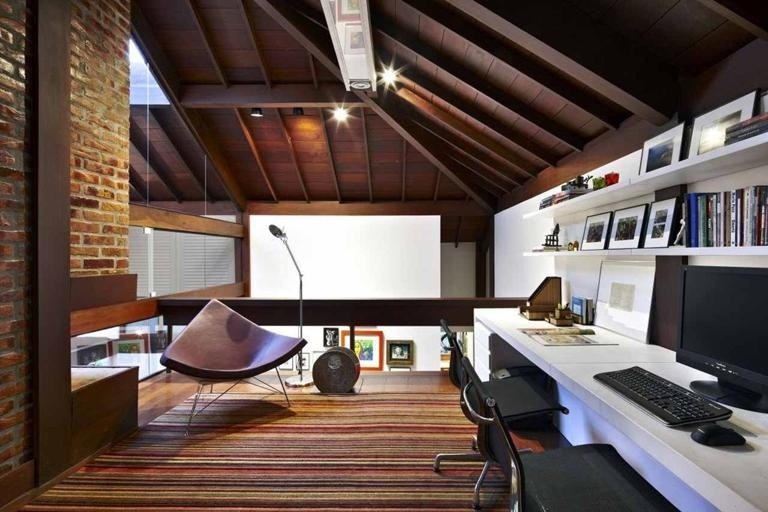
594;366;732;429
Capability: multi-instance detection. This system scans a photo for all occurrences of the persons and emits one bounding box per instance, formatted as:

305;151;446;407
391;344;409;359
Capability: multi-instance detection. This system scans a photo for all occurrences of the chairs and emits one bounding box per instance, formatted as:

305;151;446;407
159;296;308;439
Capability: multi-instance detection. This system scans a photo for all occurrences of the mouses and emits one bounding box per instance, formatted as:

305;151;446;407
692;425;746;447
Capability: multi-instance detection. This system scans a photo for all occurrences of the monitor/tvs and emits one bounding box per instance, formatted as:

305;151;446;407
676;265;768;414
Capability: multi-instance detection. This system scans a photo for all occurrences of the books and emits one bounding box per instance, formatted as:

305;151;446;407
680;186;768;247
725;110;768;146
586;297;594;325
539;187;595;208
518;328;621;346
571;296;587;324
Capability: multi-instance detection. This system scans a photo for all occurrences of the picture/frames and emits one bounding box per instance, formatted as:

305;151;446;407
578;195;679;249
109;331;150;381
637;88;761;175
342;330;415;371
